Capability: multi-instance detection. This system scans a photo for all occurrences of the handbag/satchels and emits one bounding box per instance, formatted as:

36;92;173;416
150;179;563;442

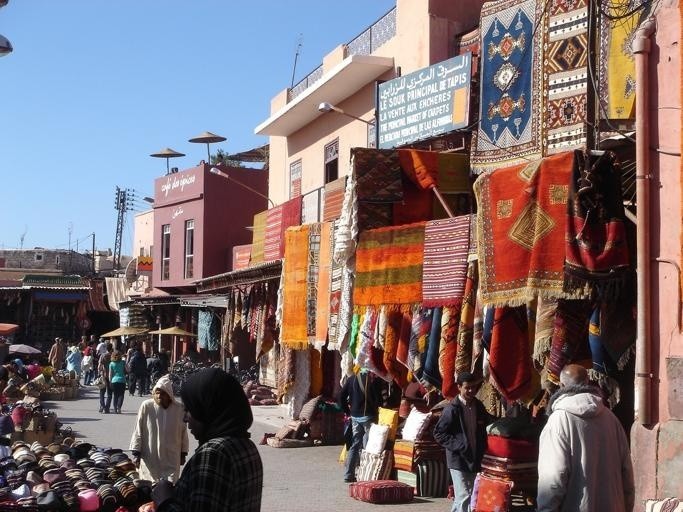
342;419;353;452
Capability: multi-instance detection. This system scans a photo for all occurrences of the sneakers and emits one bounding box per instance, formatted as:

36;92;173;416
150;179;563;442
98;406;122;414
343;477;358;483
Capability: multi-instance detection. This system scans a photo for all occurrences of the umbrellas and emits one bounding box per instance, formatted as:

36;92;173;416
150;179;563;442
100;325;149;355
150;148;186;174
188;131;228;166
148;325;199;364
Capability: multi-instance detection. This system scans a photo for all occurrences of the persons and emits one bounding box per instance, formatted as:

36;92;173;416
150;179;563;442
200;160;207;165
214;155;226;167
535;364;634;512
340;373;383;483
1;333;170;414
432;371;497;512
136;368;262;512
129;370;190;483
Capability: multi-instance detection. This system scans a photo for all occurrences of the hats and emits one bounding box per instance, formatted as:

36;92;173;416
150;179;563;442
152;374;181;407
181;368;253;436
